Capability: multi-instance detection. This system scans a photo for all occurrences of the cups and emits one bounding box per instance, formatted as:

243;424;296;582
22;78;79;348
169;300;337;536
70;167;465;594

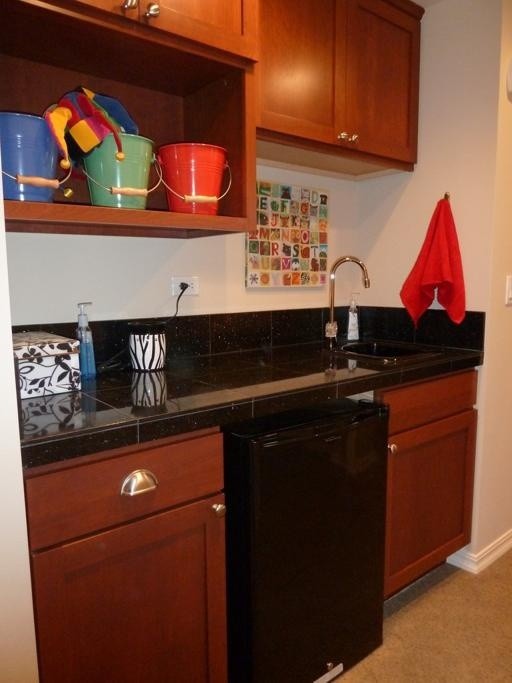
131;372;168;415
128;321;167;371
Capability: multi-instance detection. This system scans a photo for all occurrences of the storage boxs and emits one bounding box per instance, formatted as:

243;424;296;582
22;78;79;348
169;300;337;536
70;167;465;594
11;330;83;398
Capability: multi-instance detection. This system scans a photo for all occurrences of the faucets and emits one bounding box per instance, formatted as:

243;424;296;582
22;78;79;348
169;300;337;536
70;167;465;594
324;255;370;351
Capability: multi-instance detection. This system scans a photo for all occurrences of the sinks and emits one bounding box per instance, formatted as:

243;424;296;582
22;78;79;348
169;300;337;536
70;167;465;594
341;340;446;364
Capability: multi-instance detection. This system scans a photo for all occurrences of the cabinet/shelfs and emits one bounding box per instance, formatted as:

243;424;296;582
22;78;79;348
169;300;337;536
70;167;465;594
375;368;478;600
1;0;253;240
252;0;426;178
21;425;228;682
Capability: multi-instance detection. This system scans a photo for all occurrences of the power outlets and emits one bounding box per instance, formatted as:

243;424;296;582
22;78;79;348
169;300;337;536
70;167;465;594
172;276;200;294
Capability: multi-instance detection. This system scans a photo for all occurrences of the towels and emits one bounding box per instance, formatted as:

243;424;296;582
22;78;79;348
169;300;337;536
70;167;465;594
400;194;468;324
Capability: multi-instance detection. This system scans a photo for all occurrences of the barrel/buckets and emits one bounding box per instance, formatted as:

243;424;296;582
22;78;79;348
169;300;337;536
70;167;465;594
154;143;233;215
81;132;163;210
0;111;72;203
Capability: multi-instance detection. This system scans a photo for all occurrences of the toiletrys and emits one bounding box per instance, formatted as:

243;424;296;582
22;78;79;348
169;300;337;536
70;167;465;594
346;292;361;342
76;303;97;376
